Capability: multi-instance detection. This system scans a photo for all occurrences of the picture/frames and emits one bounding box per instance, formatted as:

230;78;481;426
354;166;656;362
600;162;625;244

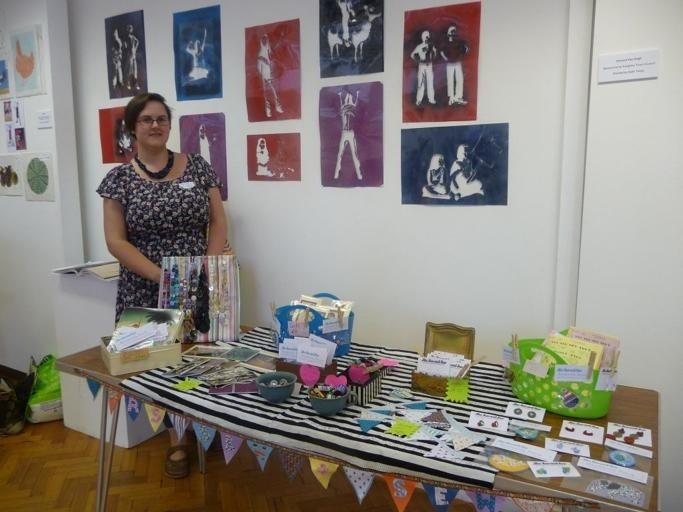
6;25;48;100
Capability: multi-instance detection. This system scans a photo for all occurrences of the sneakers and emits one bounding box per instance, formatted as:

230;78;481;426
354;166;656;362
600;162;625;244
162;435;222;478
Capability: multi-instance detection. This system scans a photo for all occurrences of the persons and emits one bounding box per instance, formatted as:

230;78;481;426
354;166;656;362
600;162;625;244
254;135;273;178
330;88;366;183
420;152;451;202
123;25;141;94
255;33;283;118
92;94;229;480
439;25;469;108
182;27;211;81
337;0;355;47
447;145;486;203
193;120;214;166
409;27;437;108
107;27;126;89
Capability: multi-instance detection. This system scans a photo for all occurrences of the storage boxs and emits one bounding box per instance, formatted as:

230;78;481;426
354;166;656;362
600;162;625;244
99;332;183;377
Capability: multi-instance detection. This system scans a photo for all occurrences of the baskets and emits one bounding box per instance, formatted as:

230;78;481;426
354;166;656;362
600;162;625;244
272;293;354;356
511;339;619;419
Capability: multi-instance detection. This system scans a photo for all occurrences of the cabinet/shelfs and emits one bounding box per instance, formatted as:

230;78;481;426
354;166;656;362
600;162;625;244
49;268;166;449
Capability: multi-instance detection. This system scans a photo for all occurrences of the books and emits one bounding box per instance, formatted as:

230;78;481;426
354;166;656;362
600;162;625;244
528;324;623;413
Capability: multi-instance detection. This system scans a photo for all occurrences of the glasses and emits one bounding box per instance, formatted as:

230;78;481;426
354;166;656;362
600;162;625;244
136;116;168;127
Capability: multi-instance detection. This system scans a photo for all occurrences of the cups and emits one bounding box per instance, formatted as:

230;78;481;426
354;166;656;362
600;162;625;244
254;371;298;403
305;386;359;416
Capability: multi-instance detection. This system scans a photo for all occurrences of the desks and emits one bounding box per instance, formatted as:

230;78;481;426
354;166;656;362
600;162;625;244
52;324;666;512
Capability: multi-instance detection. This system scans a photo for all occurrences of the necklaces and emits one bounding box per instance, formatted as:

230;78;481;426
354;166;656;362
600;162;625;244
160;255;232;343
133;148;174;180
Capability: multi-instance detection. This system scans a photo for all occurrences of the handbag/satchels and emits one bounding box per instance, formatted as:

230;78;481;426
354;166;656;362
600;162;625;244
0;364;36;434
